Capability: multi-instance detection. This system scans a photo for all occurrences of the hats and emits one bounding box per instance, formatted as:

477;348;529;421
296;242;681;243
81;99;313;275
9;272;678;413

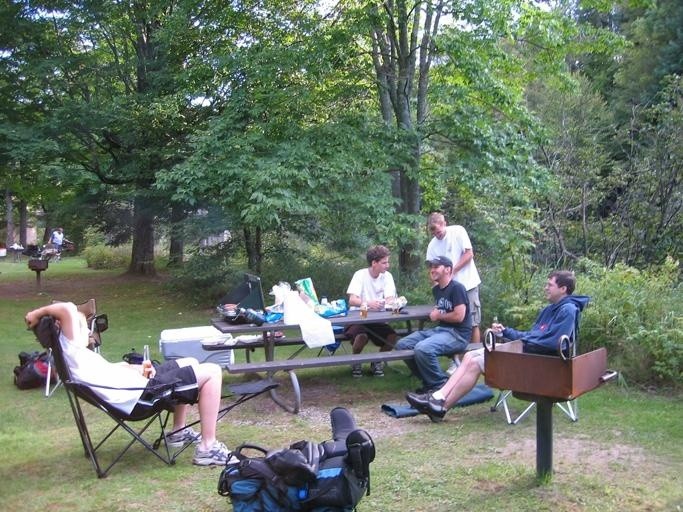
425;257;453;268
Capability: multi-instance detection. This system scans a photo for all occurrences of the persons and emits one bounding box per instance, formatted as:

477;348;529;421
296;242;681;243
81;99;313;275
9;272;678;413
344;245;399;381
24;299;241;466
405;269;587;424
394;257;472;395
425;213;483;344
48;227;74;260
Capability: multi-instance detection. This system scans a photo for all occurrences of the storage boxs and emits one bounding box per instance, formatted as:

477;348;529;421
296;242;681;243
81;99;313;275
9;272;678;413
159;326;233;371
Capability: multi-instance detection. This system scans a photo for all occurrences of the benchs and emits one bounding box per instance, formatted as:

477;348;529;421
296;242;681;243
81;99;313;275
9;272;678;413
204;327;437;363
228;344;500;413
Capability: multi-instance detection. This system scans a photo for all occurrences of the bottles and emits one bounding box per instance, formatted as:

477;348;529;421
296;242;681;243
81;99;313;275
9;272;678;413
493;316;503;343
320;296;327;305
359;293;368;320
142;345;151;378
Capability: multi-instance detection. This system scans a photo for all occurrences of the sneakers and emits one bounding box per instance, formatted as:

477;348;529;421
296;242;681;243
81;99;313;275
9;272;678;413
407;391;446;406
428;379;448;393
416;385;430;395
164;424;202;448
352;365;363;378
406;393;448;422
368;362;384;377
193;440;240;465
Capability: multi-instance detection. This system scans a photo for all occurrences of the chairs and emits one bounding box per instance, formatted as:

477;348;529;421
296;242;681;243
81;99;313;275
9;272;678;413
43;317;279;479
485;330;618;479
492;307;578;424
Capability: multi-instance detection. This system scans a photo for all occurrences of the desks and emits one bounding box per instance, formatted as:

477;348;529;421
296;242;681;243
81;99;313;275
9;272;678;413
211;302;439;387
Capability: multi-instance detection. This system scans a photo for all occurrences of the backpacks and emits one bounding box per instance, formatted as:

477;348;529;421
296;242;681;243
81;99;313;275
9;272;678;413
212;405;376;508
13;347;57;392
